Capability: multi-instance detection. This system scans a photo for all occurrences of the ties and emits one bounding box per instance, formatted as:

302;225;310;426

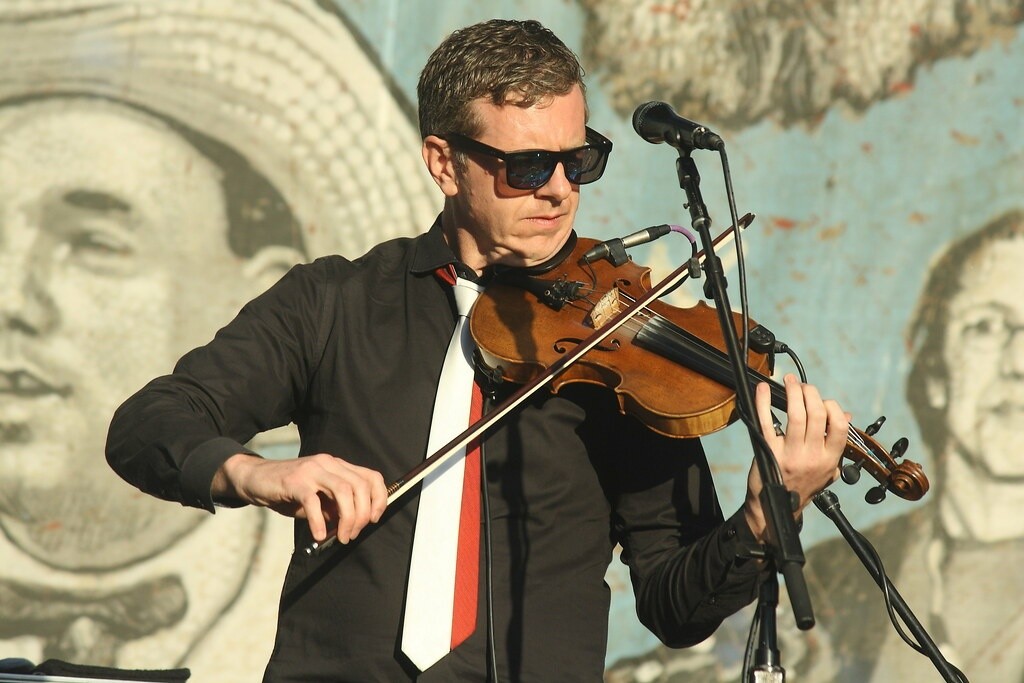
401;263;485;674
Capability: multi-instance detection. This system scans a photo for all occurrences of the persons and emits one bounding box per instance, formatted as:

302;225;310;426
104;21;852;683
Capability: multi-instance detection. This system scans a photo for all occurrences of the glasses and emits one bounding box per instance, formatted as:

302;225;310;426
448;125;613;190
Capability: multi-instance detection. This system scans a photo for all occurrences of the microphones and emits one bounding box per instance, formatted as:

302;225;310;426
632;101;724;152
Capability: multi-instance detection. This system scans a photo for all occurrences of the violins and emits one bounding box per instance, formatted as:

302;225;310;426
468;233;918;507
